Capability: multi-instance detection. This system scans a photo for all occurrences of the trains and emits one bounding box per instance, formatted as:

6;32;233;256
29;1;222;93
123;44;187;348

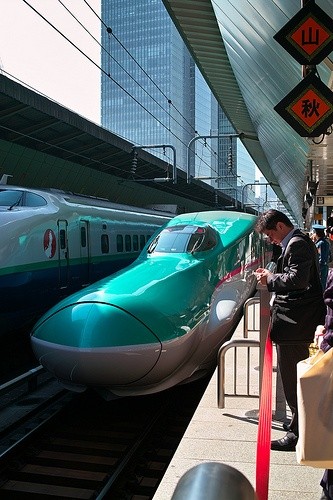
0;184;175;295
28;210;278;403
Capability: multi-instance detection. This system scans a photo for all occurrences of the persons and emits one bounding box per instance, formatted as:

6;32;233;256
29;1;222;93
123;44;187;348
303;225;333;500
256;209;321;452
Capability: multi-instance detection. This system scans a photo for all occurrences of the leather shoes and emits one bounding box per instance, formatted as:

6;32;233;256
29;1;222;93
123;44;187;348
270;437;297;451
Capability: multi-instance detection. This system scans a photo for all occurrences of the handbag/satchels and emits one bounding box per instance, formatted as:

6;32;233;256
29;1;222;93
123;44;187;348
294;347;333;468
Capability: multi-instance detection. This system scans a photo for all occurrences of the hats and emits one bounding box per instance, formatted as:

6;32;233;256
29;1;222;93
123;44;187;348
312;224;327;232
